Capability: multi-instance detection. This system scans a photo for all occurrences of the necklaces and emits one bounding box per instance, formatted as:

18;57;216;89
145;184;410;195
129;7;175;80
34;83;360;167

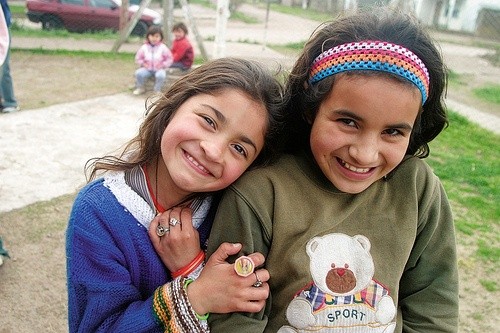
155;158;197;217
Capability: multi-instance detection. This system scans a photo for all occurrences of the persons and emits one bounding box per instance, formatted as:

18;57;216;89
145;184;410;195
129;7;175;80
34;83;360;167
0;0;21;114
65;56;284;333
203;7;459;333
130;21;194;104
0;239;9;266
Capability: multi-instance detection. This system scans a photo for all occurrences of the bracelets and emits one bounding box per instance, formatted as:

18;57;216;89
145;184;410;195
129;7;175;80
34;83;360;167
170;249;205;281
184;280;209;320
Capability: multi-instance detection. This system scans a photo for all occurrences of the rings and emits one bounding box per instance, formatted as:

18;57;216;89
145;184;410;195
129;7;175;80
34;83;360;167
234;256;256;277
156;225;170;238
253;272;264;288
168;218;181;227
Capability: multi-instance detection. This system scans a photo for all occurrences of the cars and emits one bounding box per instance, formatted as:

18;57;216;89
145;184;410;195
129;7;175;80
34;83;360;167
25;0;163;38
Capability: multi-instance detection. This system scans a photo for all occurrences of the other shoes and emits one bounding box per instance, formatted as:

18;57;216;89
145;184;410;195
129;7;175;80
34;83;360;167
133;88;145;95
3;106;19;113
